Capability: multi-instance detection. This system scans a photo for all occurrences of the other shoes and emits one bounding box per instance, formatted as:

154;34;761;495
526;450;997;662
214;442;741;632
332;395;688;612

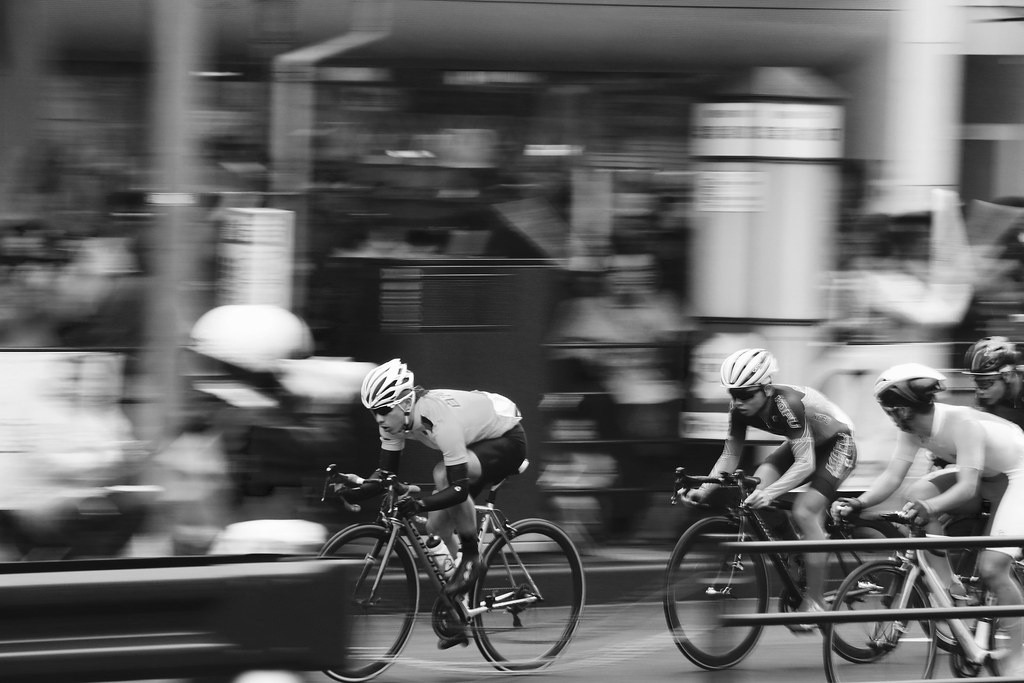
442;550;491;593
435;633;468;649
788;600;831;631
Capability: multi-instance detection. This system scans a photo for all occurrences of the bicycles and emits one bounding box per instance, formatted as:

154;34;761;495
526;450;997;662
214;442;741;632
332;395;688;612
662;467;916;672
317;463;586;683
823;505;1024;683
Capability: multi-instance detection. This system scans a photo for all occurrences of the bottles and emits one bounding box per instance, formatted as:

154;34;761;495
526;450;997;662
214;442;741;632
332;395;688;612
424;533;458;579
973;591;998;651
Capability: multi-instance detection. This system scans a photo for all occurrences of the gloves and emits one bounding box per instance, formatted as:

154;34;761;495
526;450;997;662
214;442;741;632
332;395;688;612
394;495;425;518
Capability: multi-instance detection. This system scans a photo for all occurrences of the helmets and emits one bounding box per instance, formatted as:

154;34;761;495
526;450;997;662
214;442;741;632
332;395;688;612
960;334;1024;375
359;356;418;405
873;364;950;408
720;348;780;390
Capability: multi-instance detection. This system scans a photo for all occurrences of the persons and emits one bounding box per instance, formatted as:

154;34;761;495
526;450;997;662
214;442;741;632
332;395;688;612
332;358;528;649
830;363;1024;678
672;348;856;630
0;246;1024;558
930;335;1024;469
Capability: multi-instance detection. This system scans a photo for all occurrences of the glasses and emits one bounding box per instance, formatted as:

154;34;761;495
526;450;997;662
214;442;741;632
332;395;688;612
728;388;764;401
371;391;415;416
972;377;1005;390
